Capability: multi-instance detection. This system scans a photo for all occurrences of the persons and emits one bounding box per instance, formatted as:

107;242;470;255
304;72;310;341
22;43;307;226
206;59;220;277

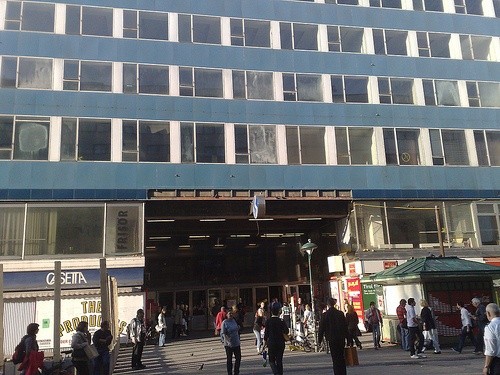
93;321;112;375
341;299;362;349
216;307;230;343
365;301;383;349
72;321;91;375
220;308;244;375
396;298;441;358
452;297;486;355
157;306;167;347
481;303;500;375
131;308;147;369
233;307;243;340
19;322;45;375
264;307;289;375
317;298;349;375
271;297;313;335
253;302;266;354
174;303;189;339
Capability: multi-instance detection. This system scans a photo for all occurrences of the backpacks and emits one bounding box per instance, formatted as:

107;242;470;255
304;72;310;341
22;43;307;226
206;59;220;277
12;335;35;365
126;318;138;339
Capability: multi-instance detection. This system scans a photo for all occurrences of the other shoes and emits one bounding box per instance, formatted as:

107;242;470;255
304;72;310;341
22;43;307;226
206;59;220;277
417;353;427;358
262;359;267;368
433;351;442;354
377;344;381;348
472;348;481;354
180;333;187;337
132;366;141;370
450;347;462;354
137;363;146;368
373;345;378;350
410;354;420;359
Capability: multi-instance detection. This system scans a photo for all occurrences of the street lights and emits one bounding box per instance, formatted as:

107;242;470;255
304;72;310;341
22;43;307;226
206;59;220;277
301;239;319;345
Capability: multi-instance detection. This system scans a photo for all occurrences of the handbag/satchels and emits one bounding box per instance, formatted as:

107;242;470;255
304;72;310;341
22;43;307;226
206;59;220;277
71;349;86;358
84;345;99;360
364;320;373;332
344;344;359;366
154;324;162;332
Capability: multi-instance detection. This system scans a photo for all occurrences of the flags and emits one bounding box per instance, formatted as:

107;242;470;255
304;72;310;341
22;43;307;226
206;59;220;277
252;196;259;219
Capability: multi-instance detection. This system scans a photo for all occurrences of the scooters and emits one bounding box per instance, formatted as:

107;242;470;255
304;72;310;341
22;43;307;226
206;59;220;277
292;335;311;352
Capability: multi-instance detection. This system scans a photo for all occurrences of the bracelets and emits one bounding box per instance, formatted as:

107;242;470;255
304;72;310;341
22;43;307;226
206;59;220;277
484;365;489;368
